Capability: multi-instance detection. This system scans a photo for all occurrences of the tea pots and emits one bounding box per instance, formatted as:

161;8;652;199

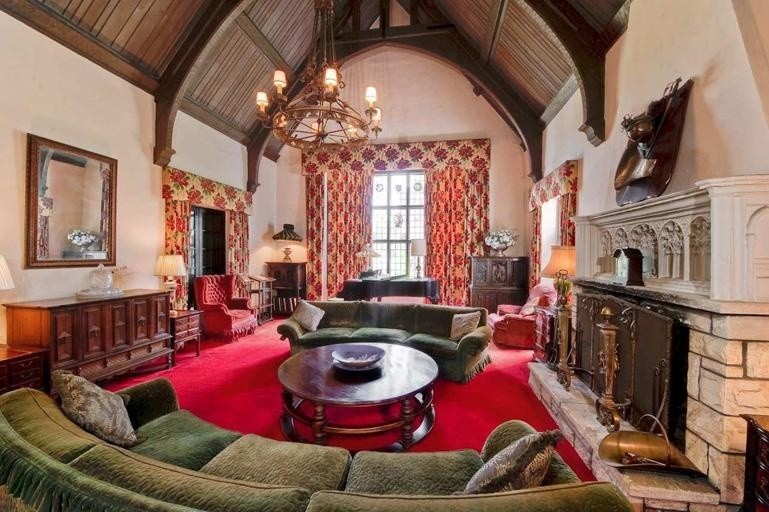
88;262;128;291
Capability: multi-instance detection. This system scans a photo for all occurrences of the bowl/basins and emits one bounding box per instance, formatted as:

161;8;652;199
331;345;385;368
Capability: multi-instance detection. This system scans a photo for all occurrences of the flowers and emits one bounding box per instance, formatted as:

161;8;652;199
67;230;96;244
485;228;515;249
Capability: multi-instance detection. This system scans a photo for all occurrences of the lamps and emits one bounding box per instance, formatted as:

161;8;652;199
411;239;426;279
541;246;576;304
272;224;302;241
153;255;186;315
256;1;382;149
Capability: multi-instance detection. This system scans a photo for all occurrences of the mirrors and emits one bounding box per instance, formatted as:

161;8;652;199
25;133;117;269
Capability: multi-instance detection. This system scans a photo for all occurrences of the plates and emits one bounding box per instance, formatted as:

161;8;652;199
331;360;386;373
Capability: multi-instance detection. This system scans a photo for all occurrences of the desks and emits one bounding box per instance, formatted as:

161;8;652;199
343;277;440;304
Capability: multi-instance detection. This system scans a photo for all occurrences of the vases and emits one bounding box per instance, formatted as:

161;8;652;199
493;245;507;257
79;243;91;252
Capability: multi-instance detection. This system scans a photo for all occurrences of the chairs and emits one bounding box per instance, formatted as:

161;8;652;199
194;274;257;341
487;284;556;349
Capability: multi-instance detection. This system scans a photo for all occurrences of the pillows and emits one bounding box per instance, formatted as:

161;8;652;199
464;429;560;494
520;296;540;315
54;372;149;449
295;300;481;340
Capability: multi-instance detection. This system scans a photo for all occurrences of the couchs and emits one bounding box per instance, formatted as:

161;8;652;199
0;376;629;511
277;299;492;383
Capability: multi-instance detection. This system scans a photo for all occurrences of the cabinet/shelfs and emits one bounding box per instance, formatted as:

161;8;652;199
468;256;529;315
0;342;46;396
170;310;204;366
535;306;577;364
266;261;308;314
0;289;174;400
249;274;276;326
